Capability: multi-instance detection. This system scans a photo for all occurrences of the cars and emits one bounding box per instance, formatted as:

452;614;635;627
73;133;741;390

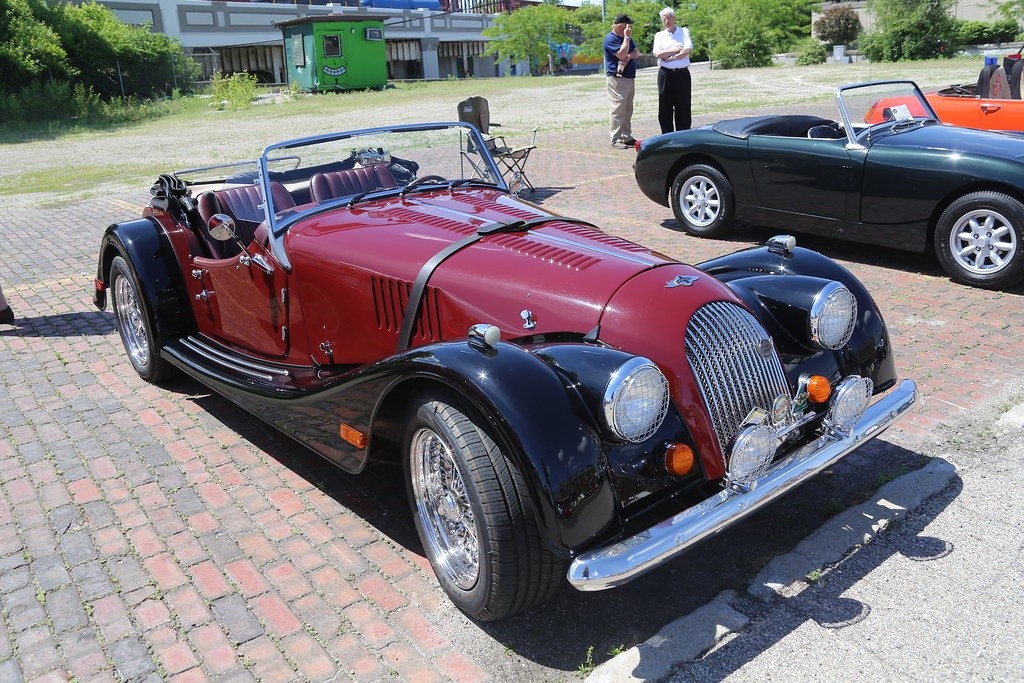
632;80;1024;289
863;83;1024;134
93;122;917;621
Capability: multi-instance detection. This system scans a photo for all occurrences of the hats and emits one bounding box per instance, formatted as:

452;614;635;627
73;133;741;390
614;13;635;24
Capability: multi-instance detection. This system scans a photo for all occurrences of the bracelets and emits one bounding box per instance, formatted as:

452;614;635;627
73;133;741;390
627;37;632;40
669;56;673;60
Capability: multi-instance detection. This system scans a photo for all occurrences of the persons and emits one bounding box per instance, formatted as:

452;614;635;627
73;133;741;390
603;14;640;149
653;7;693;134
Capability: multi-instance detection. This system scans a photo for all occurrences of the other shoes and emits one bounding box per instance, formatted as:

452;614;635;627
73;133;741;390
0;305;14;325
624;138;637;146
612;142;627;150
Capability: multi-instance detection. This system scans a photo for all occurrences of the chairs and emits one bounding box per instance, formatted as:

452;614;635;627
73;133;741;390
806;126;839;143
457;96;536;196
310;164;401;202
195;182;296;258
979;58;1024;100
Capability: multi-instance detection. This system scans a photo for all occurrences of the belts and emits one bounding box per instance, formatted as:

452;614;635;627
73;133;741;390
661;66;687;71
